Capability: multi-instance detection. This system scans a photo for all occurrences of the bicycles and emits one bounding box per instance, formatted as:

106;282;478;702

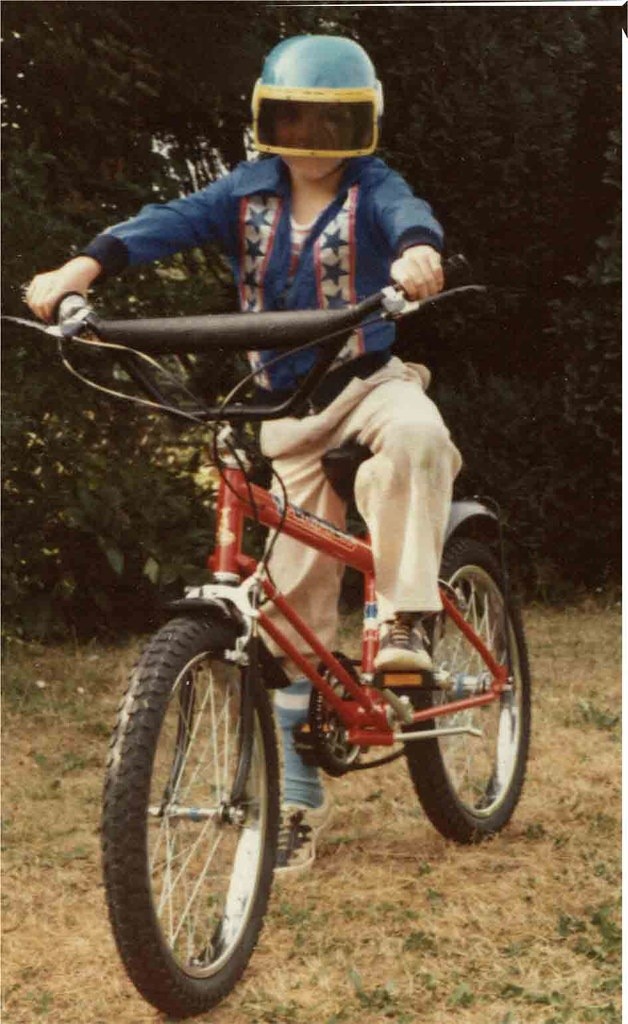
1;251;530;1016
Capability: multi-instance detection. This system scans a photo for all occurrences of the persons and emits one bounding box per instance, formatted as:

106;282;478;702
24;31;465;881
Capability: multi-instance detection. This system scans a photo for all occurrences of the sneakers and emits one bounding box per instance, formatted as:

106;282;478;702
372;612;434;672
271;790;334;876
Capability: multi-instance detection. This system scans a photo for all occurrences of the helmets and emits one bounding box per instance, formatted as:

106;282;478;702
249;34;386;159
